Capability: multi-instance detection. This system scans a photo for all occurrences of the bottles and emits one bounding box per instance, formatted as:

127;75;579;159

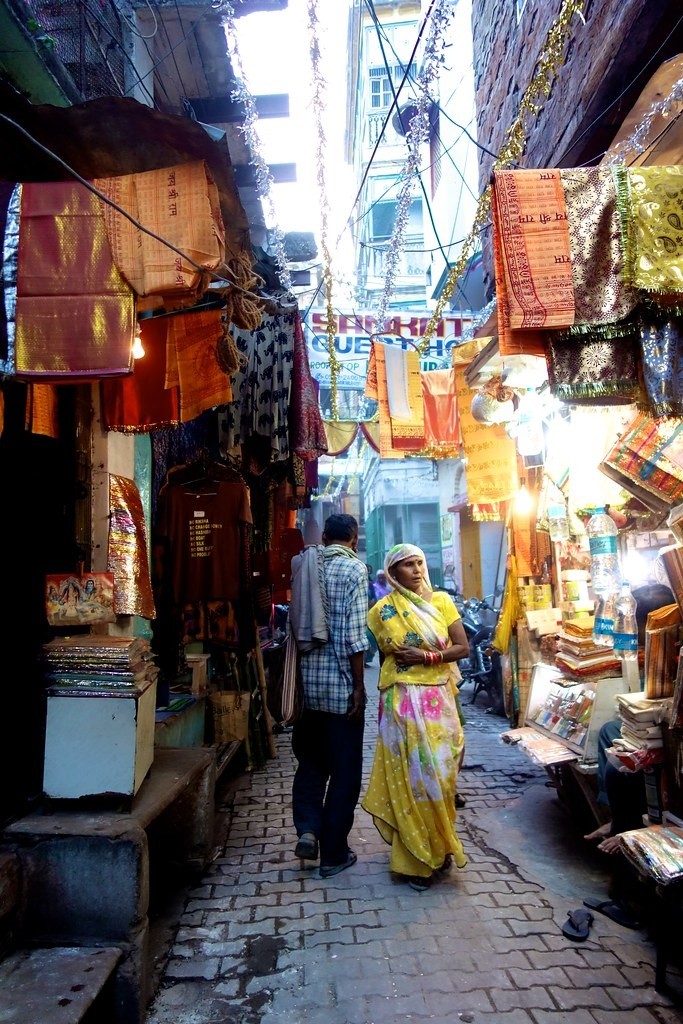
583;506;621;594
548;486;569;542
614;581;638;661
593;597;614;647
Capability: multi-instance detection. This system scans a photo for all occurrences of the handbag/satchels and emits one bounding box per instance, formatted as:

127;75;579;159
265;635;303;729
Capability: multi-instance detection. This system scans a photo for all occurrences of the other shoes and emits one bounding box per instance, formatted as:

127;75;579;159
294;833;319;860
319;847;357;877
407;875;430;890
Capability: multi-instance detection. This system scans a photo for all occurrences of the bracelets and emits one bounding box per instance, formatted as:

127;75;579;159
423;650;444;665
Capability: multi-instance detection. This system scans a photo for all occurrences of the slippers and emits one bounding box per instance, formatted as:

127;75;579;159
562;909;594;942
583;896;644;930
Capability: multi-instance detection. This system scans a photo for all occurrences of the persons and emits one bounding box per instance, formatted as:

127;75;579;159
290;514;369;877
367;543;468;892
364;564;393;668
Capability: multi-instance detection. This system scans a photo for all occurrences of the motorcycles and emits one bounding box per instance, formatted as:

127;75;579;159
433;564;510;715
